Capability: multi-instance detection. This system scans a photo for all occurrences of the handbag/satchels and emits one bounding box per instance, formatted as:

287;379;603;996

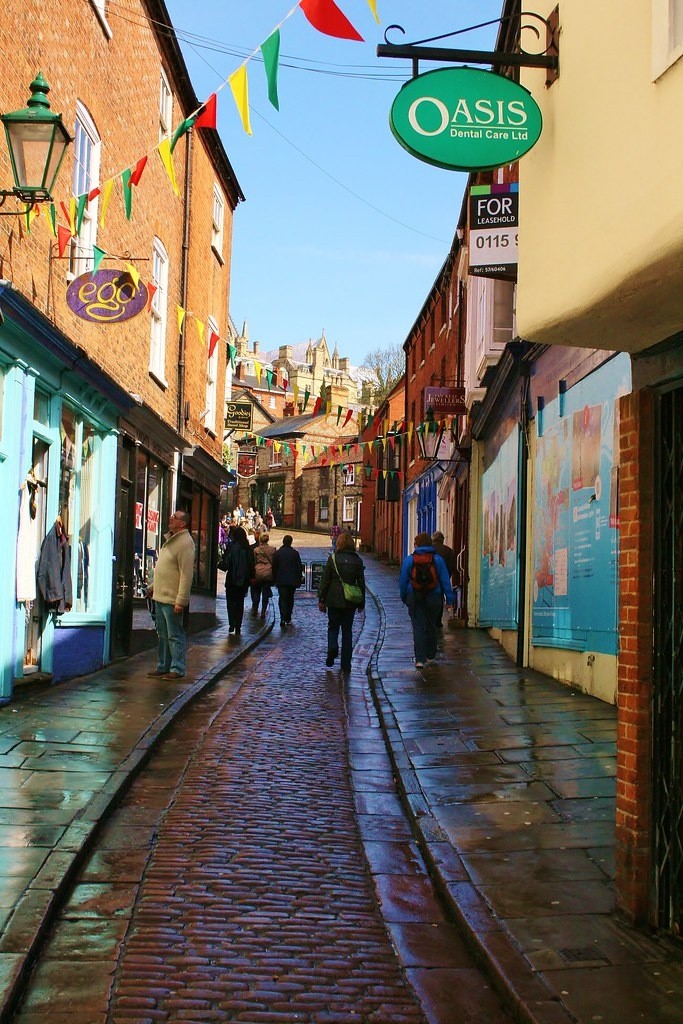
343;583;363;603
255;563;273;582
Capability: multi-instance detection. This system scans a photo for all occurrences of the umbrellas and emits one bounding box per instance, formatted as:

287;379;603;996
137;569;163;639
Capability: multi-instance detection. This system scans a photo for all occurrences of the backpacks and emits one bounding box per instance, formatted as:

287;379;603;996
410;553;437;590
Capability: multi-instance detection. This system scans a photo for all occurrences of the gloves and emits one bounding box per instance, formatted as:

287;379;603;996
358;607;364;612
319;603;326;613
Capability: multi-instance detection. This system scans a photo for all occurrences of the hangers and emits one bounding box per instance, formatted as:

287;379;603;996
56;503;64;527
20;464;42;490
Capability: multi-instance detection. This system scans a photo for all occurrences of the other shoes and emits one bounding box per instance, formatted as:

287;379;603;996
427;657;436;663
261;614;266;619
146;670;170;678
280;621;285;627
251;609;259;616
326;651;338;667
415;662;424;667
229;623;236;632
235;630;240;635
162;672;186;680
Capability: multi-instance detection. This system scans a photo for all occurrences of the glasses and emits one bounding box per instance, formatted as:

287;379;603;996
172;515;182;521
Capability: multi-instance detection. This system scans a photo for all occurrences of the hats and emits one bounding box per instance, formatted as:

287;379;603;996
240;517;246;522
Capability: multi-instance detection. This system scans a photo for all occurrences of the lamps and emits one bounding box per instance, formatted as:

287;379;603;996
0;71;75;215
415;405;470;461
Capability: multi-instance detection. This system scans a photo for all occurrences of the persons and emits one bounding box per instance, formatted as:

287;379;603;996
400;533;455;667
330;520;341;553
145;510;196;681
218;504;276;566
318;532;365;673
217;526;254;636
272;536;302;627
249;531;275;619
433;531;455;628
341;526;356;536
